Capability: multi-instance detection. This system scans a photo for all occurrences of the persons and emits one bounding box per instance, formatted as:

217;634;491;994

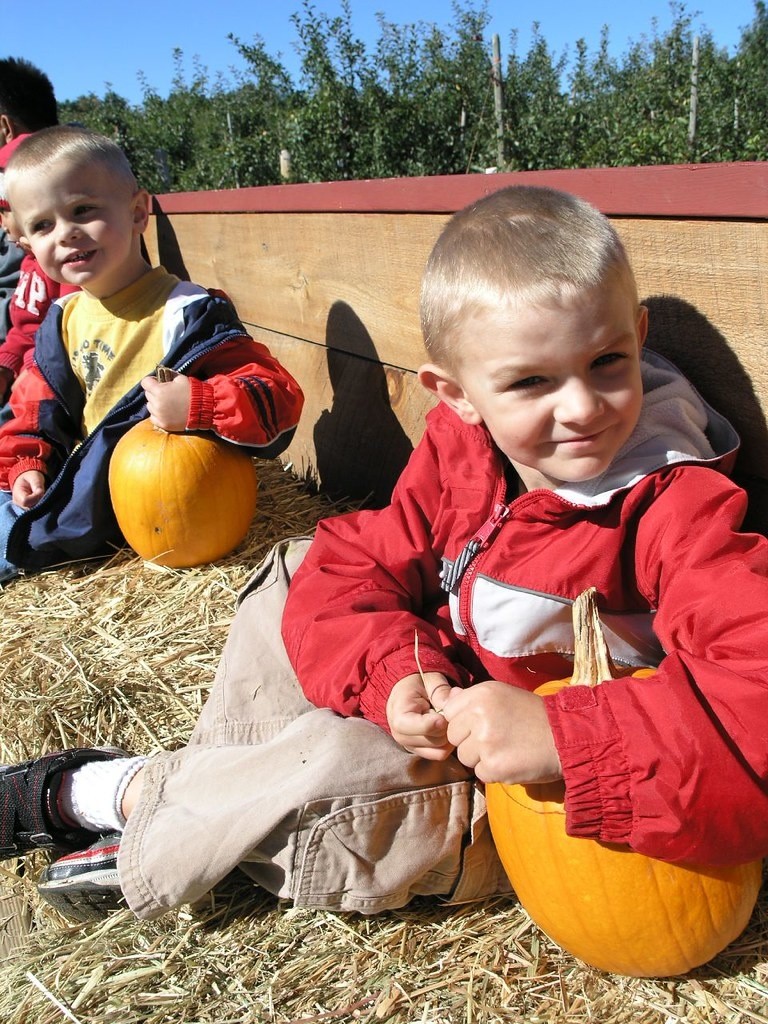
0;56;58;344
0;124;305;582
0;188;768;923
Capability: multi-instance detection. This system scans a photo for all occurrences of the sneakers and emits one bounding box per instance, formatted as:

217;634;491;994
0;746;132;861
37;830;130;924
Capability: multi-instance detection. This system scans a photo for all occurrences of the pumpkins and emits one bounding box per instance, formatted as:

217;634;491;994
109;367;256;569
485;588;764;978
